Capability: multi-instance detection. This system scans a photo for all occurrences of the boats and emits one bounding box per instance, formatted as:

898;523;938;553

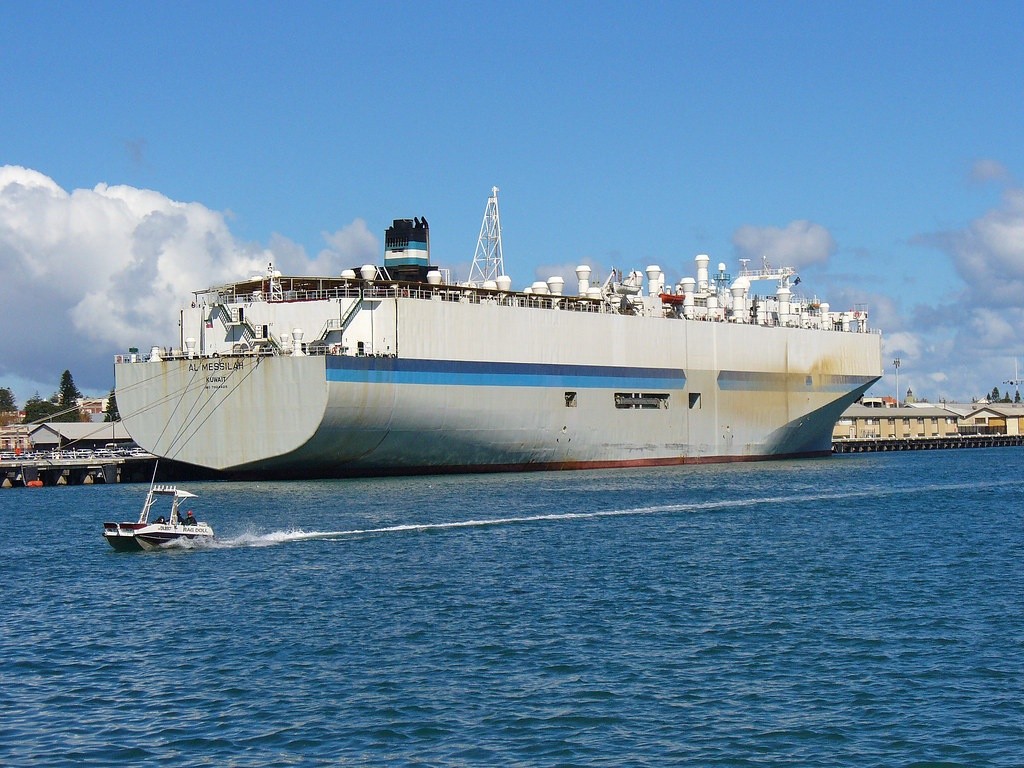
115;188;883;477
101;486;217;553
